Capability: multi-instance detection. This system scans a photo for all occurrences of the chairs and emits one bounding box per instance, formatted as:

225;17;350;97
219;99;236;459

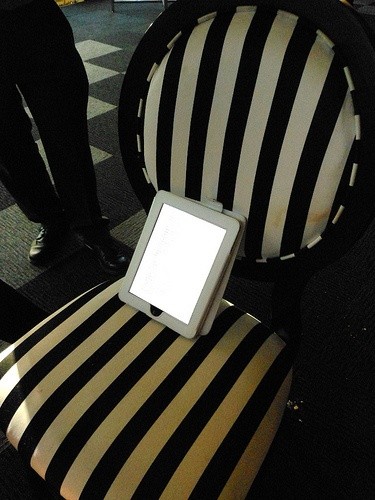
0;0;375;500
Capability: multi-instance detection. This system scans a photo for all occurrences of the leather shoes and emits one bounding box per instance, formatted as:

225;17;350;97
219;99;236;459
71;228;125;277
29;217;71;265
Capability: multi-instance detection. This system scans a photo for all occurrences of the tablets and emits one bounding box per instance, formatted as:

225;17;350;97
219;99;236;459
118;190;247;340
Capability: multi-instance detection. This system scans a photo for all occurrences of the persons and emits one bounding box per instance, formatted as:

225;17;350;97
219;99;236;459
0;1;129;273
